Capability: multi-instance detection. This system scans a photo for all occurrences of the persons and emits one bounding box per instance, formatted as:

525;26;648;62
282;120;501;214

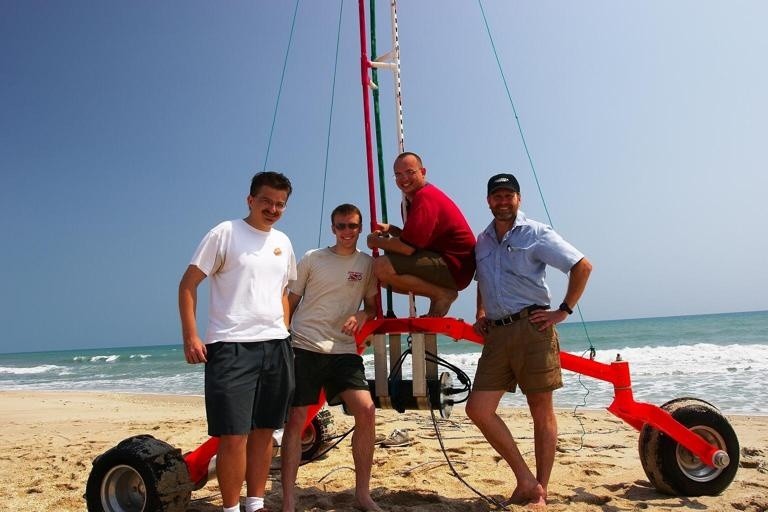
280;204;383;512
178;171;297;512
367;152;477;317
466;175;592;509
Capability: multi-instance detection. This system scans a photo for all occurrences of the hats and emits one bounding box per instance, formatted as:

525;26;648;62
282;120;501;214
486;173;521;195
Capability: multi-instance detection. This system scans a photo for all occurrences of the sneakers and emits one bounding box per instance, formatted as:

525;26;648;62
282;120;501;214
375;429;415;448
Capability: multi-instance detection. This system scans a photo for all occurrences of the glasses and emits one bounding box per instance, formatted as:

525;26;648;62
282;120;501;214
333;222;361;230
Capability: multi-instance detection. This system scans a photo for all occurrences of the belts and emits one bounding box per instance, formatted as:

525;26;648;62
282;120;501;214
487;303;551;327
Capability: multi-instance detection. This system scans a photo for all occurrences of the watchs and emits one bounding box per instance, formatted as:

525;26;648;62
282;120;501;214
560;304;573;314
